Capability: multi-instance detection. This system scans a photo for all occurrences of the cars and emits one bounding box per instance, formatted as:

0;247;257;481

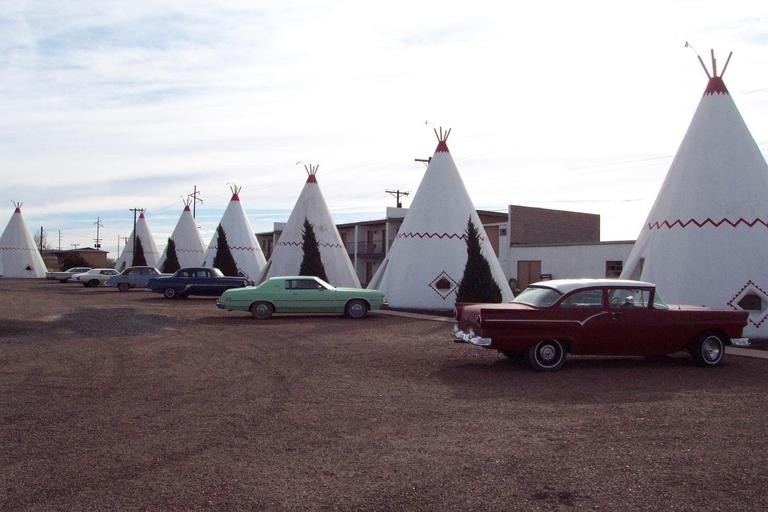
72;269;121;287
217;276;385;319
456;279;750;372
152;267;254;299
107;266;175;292
46;267;91;282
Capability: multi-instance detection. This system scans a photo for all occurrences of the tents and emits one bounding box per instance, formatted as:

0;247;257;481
113;212;162;276
0;206;51;279
371;139;519;313
254;174;362;296
609;77;768;345
157;204;206;270
202;193;268;285
